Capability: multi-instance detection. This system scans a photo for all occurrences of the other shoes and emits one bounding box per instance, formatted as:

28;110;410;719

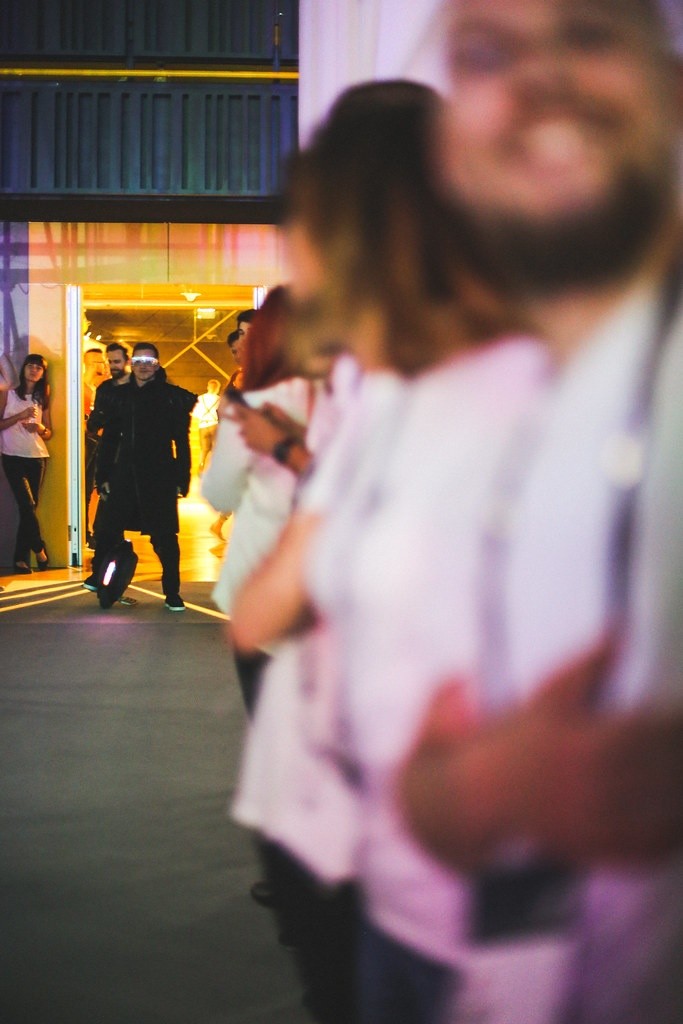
165;595;185;612
36;540;48;571
15;561;32;574
82;578;98;590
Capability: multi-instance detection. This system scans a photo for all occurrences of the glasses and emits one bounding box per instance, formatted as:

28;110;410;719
131;357;158;367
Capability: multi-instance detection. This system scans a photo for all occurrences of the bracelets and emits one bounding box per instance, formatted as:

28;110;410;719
39;427;48;436
273;437;296;463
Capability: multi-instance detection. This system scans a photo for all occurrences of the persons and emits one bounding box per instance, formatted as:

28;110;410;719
192;379;221;477
82;342;199;610
202;0;683;1024
0;354;53;574
85;349;110;415
87;343;135;433
